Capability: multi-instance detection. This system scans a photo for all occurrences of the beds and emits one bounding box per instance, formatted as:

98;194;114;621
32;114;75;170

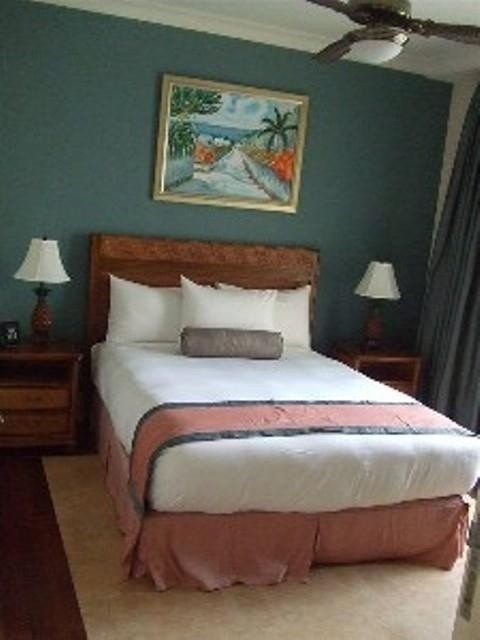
88;232;480;592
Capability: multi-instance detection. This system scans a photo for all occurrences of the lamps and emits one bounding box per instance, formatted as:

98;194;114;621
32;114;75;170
351;262;402;342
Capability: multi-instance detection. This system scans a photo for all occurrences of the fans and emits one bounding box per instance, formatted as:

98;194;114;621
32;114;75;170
304;0;480;68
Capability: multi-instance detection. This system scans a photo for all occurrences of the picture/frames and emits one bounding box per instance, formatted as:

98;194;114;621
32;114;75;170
151;71;311;216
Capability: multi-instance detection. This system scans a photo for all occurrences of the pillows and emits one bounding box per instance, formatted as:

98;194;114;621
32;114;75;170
106;272;183;343
215;282;312;354
12;236;72;337
175;273;277;355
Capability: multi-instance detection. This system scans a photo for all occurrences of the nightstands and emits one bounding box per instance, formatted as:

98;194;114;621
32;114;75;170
0;351;83;451
338;348;422;399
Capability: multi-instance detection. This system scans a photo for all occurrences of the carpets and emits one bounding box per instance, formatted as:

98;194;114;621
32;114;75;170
40;455;480;640
